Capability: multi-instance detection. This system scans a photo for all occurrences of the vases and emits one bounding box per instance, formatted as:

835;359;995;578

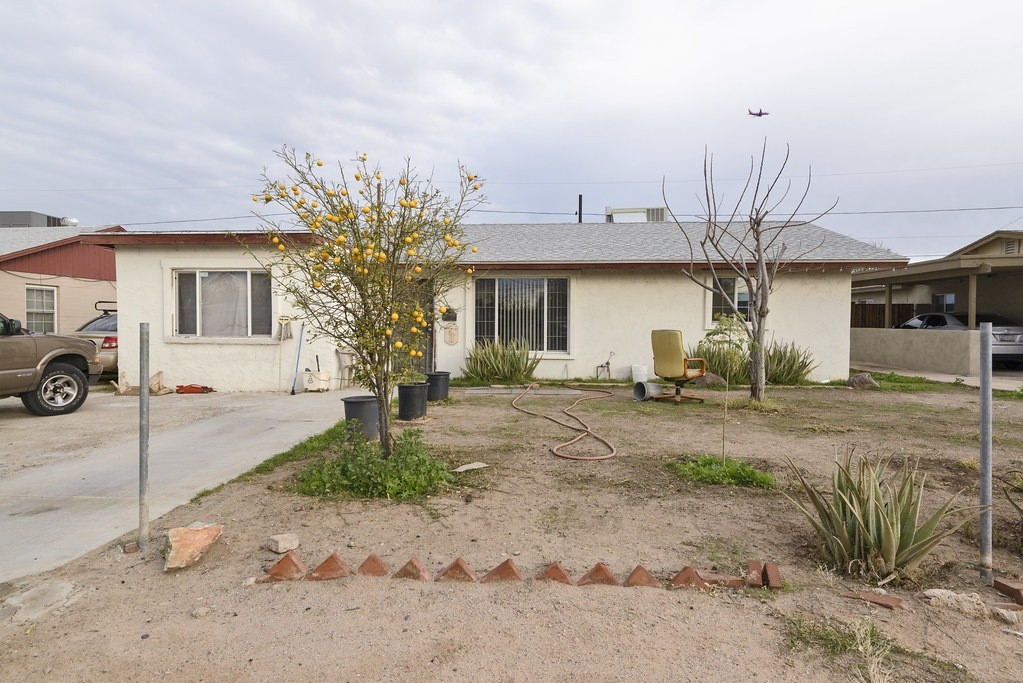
342;395;380;442
422;371;451;401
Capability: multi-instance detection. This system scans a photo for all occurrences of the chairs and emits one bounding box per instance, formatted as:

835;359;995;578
650;330;707;405
336;347;370;391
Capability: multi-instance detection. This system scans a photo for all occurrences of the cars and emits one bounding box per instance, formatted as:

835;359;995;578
67;301;121;380
0;309;103;415
888;312;1023;371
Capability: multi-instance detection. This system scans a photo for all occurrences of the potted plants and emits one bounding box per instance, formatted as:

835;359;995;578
394;336;430;420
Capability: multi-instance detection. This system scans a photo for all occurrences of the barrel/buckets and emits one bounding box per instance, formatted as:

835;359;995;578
632;380;664;401
340;395;379;441
396;382;430;422
631;365;647;382
423;371;451;402
